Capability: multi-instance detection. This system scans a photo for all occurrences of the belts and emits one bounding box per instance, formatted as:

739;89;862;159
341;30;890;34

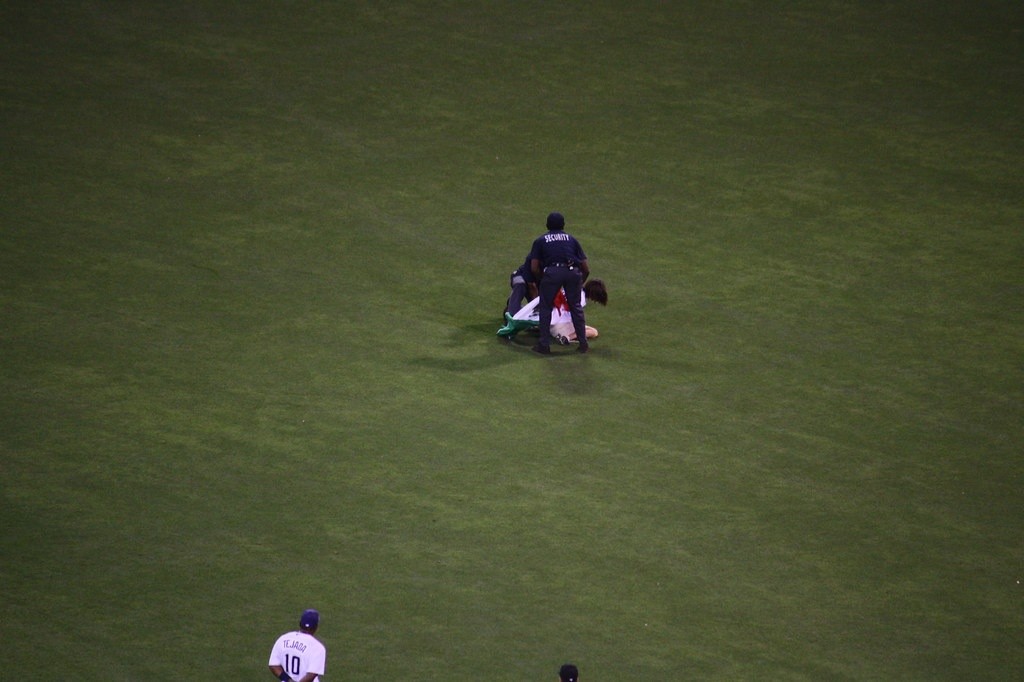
548;262;576;267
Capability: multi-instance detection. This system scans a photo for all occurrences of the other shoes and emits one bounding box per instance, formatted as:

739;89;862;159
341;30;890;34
531;343;550;354
576;344;589;353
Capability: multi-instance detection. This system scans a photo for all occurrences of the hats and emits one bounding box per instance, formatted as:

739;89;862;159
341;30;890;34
547;213;565;227
302;610;318;627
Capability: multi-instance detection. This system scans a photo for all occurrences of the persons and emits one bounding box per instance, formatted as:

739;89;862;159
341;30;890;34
269;609;326;682
550;278;608;346
528;212;589;354
504;253;538;328
558;663;578;682
